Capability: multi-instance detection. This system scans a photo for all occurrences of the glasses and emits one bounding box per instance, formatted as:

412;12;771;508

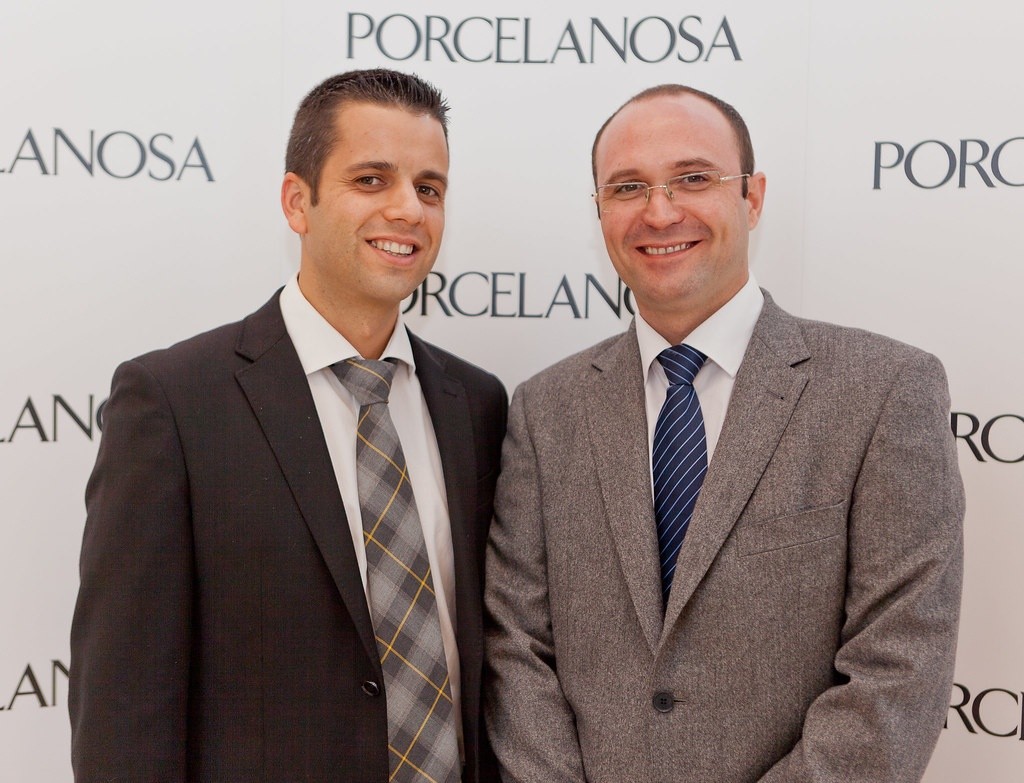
591;170;750;215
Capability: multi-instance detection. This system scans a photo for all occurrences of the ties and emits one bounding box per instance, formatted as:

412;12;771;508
327;356;461;783
653;344;709;622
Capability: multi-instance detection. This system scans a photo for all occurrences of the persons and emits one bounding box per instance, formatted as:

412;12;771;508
482;85;966;783
67;69;511;783
